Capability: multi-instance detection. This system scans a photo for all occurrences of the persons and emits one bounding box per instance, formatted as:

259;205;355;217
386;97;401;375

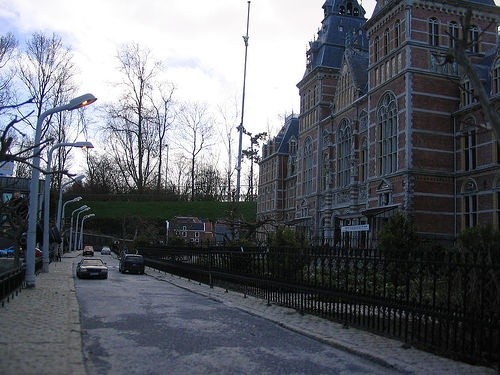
57;246;62;262
135;248;140;255
121;249;126;257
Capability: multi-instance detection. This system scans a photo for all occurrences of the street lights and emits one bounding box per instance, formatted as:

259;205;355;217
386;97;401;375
59;197;82;256
68;205;87;253
53;175;85;262
26;93;97;288
79;214;95;249
81;215;89;250
73;207;91;252
41;142;93;274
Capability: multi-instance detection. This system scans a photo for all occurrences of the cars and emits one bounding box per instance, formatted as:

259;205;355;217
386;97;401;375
76;258;108;279
118;254;144;274
0;245;16;259
101;247;111;256
14;248;43;259
82;246;94;256
